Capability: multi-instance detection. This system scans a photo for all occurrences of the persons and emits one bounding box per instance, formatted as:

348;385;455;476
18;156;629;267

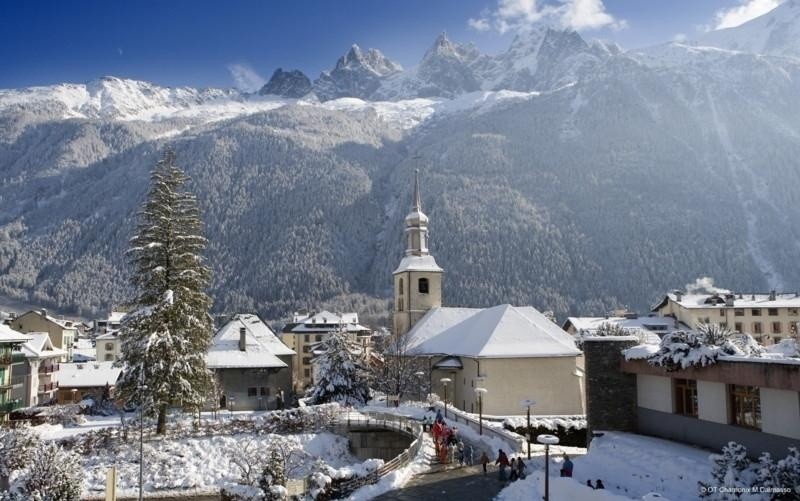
516;457;527;479
494;449;510;483
508;458;518;481
596;480;604;490
421;409;474;467
561;455;573;477
586;480;594;490
480;452;490;474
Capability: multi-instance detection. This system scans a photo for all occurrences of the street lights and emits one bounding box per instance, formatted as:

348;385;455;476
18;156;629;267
537;435;560;501
415;372;425;401
520;400;536;461
440;378;451;418
474;387;487;436
